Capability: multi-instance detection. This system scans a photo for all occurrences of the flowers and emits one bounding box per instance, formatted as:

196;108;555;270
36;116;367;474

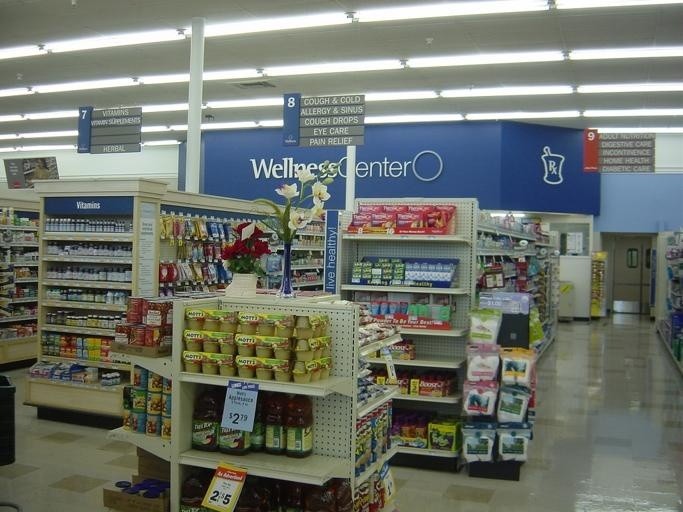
252;161;343;245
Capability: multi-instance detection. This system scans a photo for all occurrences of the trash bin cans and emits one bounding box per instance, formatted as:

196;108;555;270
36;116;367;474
0;375;15;467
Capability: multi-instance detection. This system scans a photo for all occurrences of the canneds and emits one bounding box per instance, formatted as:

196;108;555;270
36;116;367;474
115;288;226;440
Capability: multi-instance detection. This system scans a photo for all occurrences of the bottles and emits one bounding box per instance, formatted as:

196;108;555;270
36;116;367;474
44;218;133;305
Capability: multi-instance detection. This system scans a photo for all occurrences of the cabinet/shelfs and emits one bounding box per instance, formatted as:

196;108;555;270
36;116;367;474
21;177;327;420
0;181;40;366
336;198;561;474
106;292;402;512
